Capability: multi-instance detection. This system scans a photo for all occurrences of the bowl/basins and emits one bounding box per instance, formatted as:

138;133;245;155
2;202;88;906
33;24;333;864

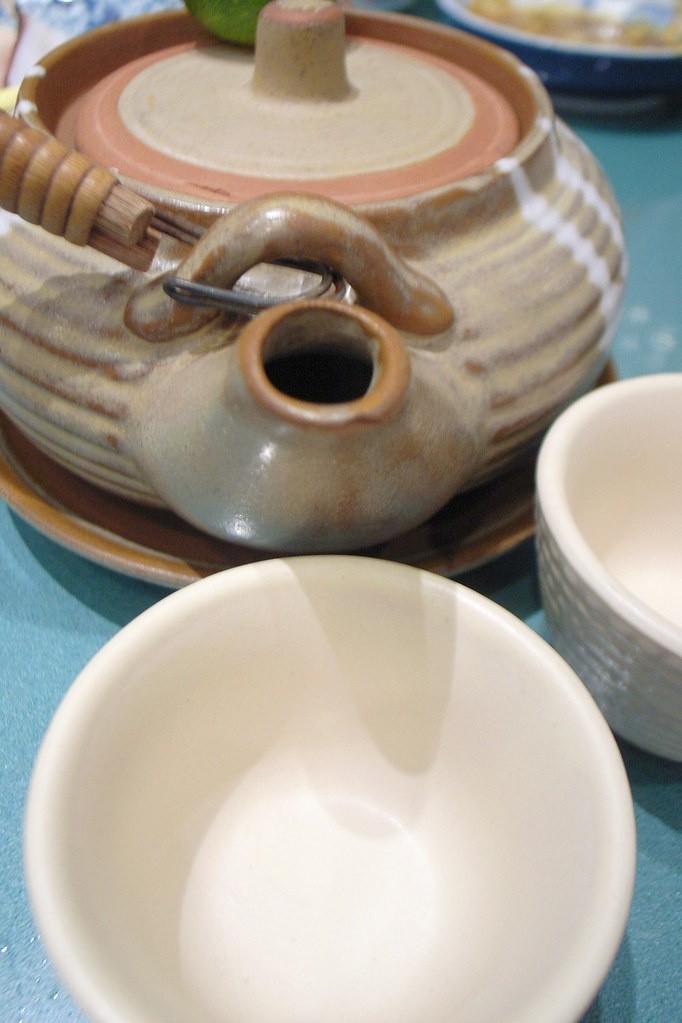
434;0;682;99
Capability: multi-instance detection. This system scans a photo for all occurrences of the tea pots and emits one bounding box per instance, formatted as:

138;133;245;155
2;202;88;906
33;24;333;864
0;0;629;554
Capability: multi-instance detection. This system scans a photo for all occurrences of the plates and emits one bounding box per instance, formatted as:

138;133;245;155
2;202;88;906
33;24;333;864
0;358;623;588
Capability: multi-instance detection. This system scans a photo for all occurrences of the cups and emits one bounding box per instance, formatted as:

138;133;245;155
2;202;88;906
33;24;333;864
24;556;636;1022
534;372;682;762
